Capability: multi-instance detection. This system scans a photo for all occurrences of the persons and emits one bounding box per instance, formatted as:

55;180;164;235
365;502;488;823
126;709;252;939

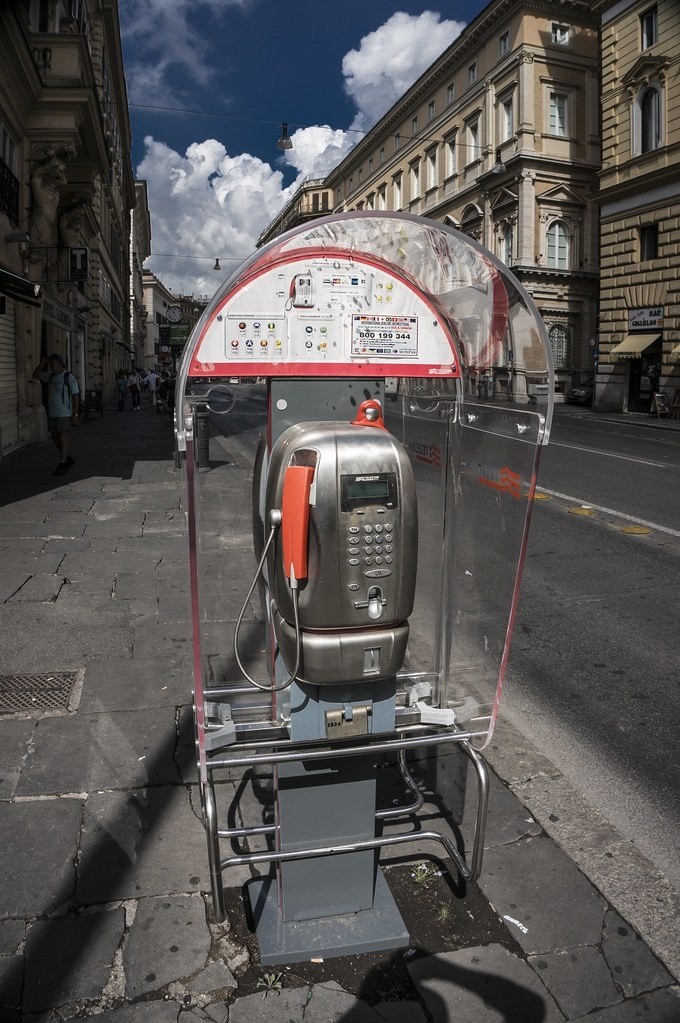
33;353;80;465
115;373;127;411
127;367;143;409
143;369;161;406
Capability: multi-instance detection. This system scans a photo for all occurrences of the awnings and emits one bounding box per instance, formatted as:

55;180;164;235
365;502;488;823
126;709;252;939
609;335;661;358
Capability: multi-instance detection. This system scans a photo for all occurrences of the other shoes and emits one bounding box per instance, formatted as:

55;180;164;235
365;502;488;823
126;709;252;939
50;455;77;469
134;407;137;411
52;462;75;474
137;406;141;410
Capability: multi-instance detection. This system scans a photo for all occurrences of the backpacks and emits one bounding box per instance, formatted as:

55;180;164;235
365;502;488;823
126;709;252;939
49;372;82;419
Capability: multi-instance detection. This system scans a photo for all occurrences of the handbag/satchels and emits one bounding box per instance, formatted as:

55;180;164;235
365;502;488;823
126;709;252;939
128;384;138;391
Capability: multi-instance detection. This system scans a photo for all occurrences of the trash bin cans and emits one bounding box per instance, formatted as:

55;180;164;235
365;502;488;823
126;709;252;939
174;391;212;468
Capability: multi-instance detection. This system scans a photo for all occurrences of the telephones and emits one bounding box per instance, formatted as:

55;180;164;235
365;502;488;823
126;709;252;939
258;399;420;689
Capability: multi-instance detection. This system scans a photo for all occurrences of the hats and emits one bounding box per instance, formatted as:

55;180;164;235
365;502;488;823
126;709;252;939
48;354;65;368
